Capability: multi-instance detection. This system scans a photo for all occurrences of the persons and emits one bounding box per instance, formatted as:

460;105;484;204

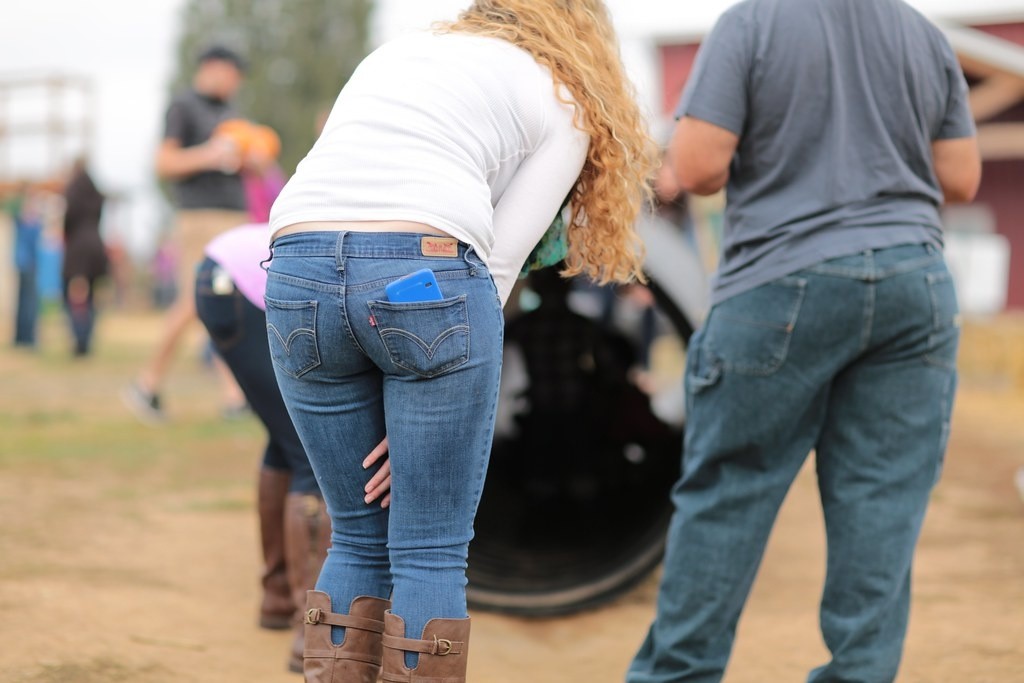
13;49;664;626
259;0;654;683
649;0;981;683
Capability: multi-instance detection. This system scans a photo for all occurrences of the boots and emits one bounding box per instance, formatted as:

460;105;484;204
284;492;335;672
258;464;296;628
302;587;391;683
377;607;471;683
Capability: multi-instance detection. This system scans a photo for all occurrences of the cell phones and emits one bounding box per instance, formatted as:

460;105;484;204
385;268;444;303
